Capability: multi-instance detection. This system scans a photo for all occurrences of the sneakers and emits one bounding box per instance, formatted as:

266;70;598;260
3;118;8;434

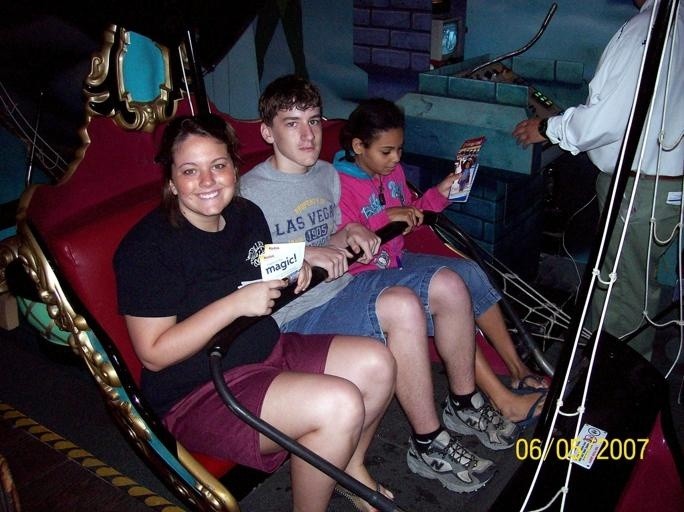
406;430;501;496
443;384;524;452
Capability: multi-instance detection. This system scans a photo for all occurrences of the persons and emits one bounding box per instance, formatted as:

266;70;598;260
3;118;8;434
108;109;400;510
457;159;472;192
225;72;523;494
509;2;682;368
331;94;556;431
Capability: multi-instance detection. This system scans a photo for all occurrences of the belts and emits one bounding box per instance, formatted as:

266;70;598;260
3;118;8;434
626;170;684;183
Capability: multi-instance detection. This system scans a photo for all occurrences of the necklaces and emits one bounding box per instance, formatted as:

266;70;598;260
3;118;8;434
356;163;387;206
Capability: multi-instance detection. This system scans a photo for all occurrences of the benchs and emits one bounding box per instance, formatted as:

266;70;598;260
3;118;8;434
16;18;480;512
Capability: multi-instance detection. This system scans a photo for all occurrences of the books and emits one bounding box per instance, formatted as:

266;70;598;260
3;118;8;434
445;162;479;206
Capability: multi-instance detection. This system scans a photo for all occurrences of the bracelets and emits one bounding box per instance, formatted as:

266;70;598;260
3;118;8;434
537;117;548;140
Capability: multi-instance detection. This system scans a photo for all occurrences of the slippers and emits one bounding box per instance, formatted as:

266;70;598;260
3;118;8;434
508;369;551;393
334;474;400;512
496;387;551;424
